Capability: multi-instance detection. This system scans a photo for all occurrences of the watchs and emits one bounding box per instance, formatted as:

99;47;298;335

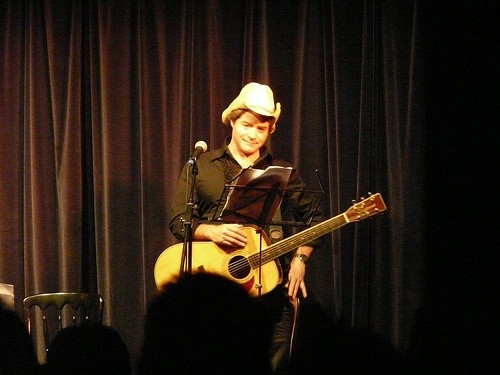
293;253;308;262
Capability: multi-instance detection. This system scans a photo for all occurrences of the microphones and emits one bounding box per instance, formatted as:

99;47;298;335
187;140;208;167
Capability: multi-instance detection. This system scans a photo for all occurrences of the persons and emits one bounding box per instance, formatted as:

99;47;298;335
167;82;326;375
0;272;500;375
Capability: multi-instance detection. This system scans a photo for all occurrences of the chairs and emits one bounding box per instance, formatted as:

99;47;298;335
23;292;103;365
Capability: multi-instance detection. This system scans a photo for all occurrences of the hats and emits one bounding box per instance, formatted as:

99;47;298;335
222;82;281;134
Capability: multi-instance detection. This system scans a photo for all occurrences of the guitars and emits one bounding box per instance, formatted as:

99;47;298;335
153;190;389;303
